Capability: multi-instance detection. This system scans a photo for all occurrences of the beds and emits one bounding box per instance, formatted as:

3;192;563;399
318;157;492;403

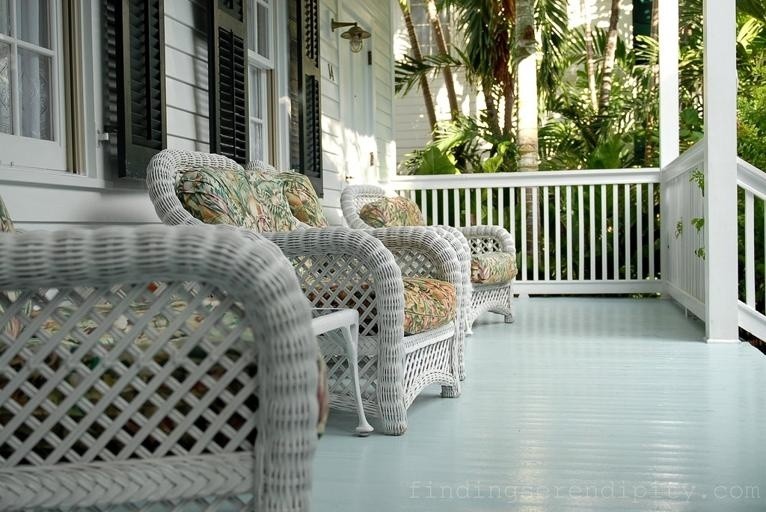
308;307;374;438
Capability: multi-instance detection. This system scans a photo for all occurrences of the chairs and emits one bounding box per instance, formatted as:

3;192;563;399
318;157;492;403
339;185;518;336
146;147;462;436
0;193;321;510
